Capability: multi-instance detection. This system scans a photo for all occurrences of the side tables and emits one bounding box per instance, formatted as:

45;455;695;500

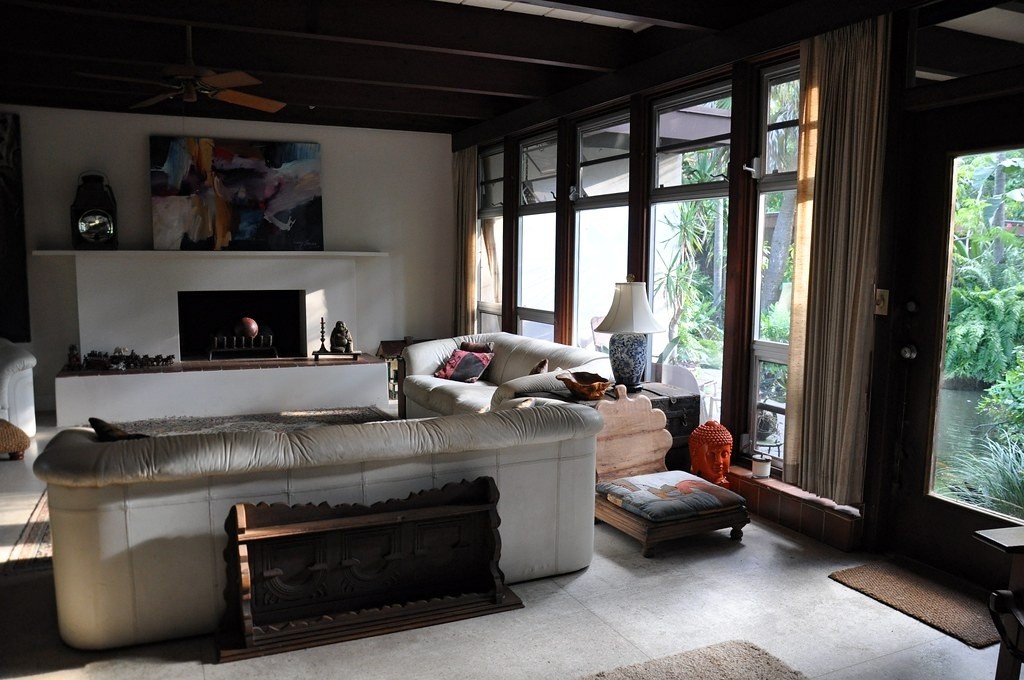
514;380;700;472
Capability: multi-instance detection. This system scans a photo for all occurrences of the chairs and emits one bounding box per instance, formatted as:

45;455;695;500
594;385;751;559
591;316;611;351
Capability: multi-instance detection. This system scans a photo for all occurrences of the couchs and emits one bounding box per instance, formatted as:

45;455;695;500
32;397;604;652
0;337;37;438
402;332;614;419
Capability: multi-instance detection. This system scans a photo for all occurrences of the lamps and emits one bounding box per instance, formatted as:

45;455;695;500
593;274;667;394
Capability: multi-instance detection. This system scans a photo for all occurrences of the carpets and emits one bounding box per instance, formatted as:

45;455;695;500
579;639;808;680
0;404;397;578
828;558;1002;650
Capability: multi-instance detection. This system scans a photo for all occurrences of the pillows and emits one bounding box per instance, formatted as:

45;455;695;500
460;341;494;375
87;416;150;443
529;358;549;375
434;349;494;383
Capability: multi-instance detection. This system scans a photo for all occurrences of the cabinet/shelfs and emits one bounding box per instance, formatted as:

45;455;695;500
54;351;389;428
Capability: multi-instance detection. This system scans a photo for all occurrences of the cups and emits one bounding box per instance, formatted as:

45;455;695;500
752;454;772;478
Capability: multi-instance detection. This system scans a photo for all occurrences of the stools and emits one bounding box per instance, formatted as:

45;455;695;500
757;438;782;457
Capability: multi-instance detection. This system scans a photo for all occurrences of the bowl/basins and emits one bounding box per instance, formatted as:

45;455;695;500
555;372;611;399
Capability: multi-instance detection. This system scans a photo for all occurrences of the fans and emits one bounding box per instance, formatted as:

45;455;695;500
70;24;287;114
